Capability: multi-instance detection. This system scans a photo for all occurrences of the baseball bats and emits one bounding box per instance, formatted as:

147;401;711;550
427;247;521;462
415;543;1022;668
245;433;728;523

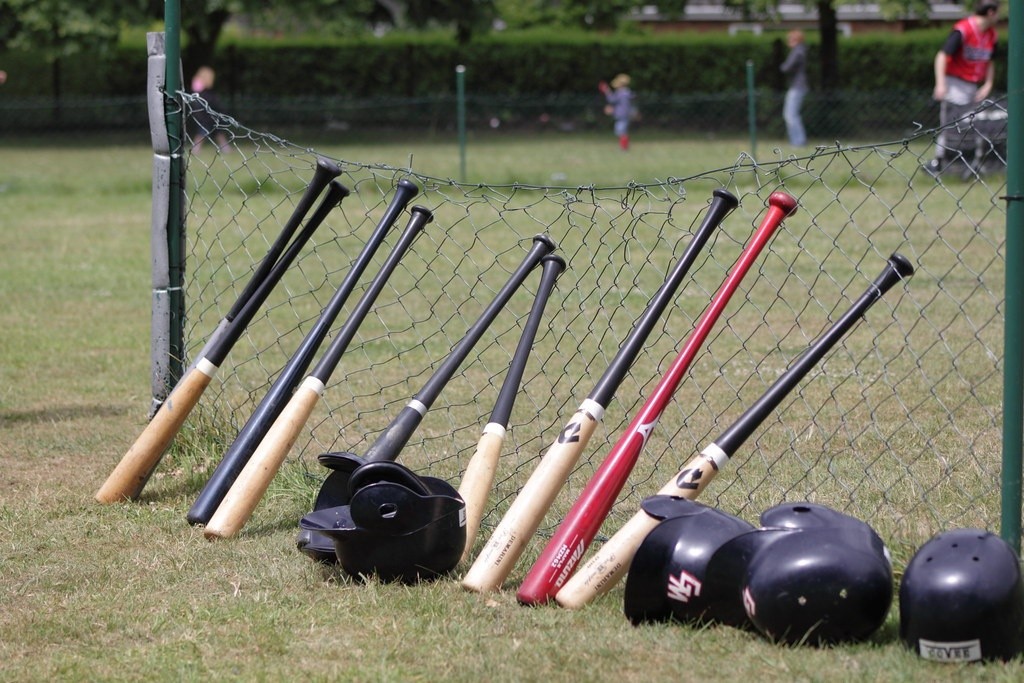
452;253;569;567
92;152;355;508
360;232;558;468
552;251;918;614
458;186;742;597
512;187;801;610
184;177;435;543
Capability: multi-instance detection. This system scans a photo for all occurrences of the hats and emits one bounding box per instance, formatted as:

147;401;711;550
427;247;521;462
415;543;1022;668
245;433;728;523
610;73;630;88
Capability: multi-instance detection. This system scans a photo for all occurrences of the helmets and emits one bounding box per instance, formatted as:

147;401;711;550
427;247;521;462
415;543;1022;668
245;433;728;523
699;502;894;650
624;496;758;631
295;451;467;586
898;529;1024;666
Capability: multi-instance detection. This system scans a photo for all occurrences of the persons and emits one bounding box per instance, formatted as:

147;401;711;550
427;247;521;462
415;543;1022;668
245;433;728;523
182;21;230;155
780;30;809;146
929;0;1000;173
371;0;419;30
600;74;632;149
